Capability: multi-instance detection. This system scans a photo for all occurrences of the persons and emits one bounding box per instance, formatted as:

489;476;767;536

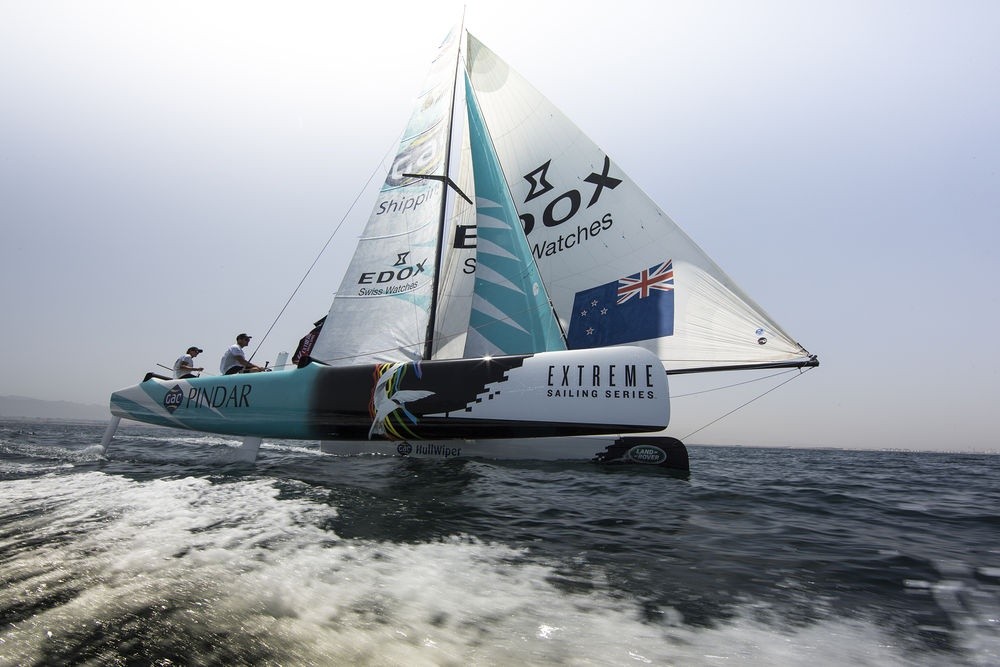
220;332;264;375
174;346;204;379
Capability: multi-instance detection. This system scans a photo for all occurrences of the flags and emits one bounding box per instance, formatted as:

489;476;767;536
566;259;674;349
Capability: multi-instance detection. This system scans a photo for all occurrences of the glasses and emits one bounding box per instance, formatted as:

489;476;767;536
245;338;250;342
195;351;199;354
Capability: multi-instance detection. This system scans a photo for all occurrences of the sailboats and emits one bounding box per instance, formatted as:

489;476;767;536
96;9;821;482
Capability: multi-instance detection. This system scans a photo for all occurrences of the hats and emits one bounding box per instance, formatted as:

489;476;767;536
188;347;203;352
237;334;252;341
